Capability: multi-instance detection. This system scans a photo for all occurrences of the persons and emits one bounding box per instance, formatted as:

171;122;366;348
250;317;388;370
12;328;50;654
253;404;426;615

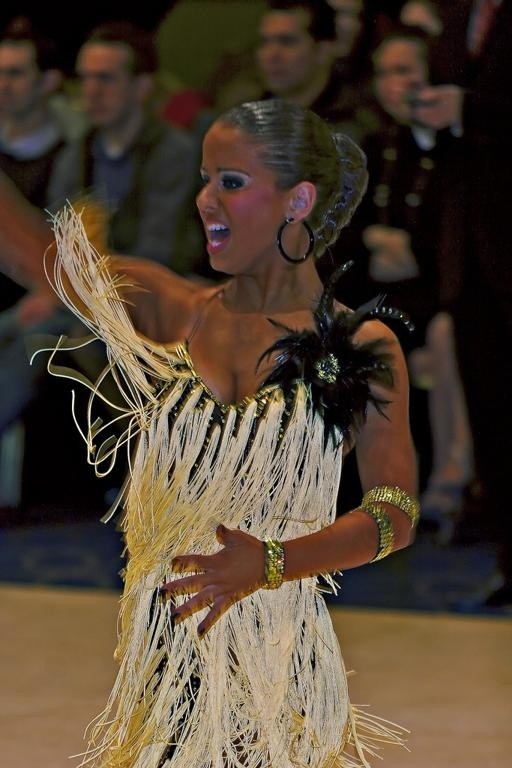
0;0;512;548
0;99;418;768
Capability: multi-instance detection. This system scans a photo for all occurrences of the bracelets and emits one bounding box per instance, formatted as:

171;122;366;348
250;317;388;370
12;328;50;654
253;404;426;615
353;507;394;564
260;540;285;590
